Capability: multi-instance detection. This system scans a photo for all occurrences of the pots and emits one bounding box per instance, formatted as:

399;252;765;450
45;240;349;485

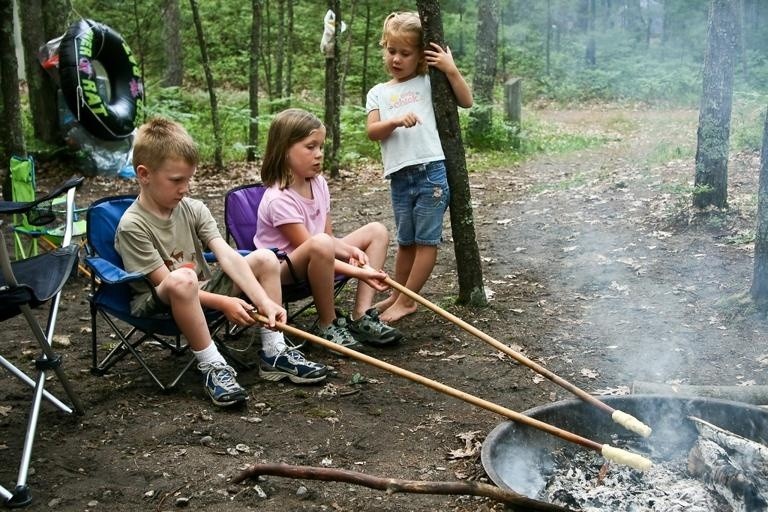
479;392;768;512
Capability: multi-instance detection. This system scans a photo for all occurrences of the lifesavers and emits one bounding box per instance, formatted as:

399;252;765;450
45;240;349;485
60;18;140;140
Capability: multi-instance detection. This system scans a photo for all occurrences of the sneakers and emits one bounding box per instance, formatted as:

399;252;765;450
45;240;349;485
311;318;365;357
258;347;329;385
346;309;404;347
196;362;249;408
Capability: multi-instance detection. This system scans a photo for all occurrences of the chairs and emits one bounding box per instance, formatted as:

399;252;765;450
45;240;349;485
86;195;255;394
223;183;353;337
0;154;87;505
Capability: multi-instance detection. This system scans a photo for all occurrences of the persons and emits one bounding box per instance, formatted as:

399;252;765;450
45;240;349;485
111;113;328;408
365;10;474;325
252;107;405;356
